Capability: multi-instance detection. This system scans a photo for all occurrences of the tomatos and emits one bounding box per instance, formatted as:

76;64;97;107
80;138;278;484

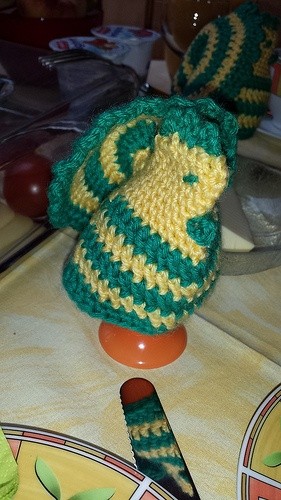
3;153;56;219
99;316;188;371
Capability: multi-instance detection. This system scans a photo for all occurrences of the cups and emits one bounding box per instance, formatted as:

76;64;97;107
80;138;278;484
49;37;131;65
92;25;161;96
